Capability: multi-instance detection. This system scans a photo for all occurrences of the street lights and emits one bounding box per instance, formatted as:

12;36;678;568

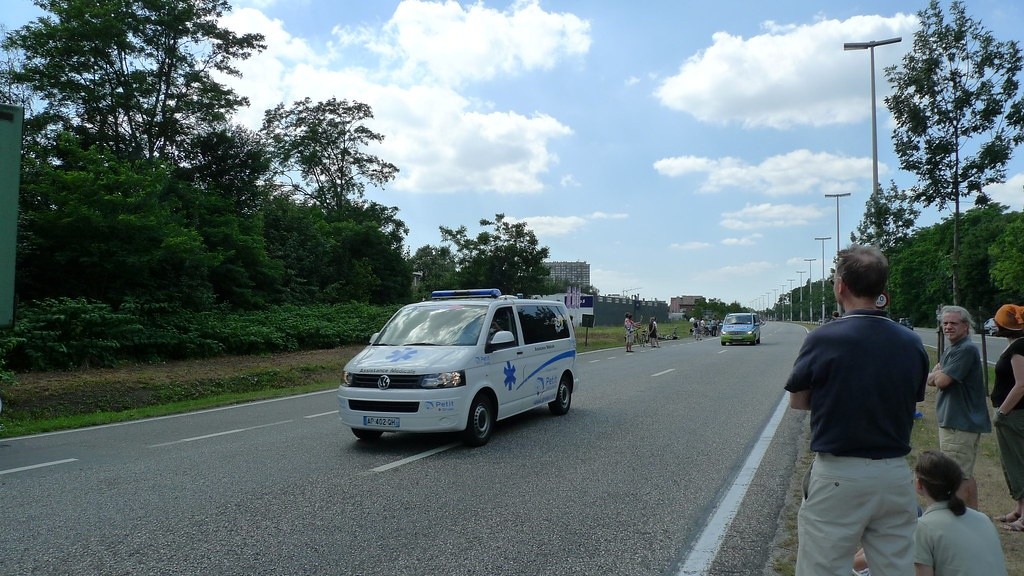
795;270;806;321
803;259;816;323
824;193;852;317
844;37;903;252
814;237;831;326
760;279;796;323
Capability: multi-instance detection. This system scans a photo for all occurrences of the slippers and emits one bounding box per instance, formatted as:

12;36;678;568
993;511;1021;522
1003;516;1024;531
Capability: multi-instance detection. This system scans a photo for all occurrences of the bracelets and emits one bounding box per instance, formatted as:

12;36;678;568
996;407;1004;416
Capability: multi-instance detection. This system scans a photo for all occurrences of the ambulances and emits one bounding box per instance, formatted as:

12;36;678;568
336;288;579;448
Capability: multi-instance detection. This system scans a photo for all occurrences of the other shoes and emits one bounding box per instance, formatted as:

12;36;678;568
626;350;634;352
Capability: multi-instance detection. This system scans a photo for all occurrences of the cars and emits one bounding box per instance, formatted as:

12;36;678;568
984;317;999;337
898;318;913;331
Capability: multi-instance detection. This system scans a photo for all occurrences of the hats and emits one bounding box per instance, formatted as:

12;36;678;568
995;304;1024;330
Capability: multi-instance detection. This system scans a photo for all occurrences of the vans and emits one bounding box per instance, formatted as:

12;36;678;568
719;312;761;345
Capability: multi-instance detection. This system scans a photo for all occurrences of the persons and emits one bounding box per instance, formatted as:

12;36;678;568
689;316;724;341
829;311;839;323
784;244;931;576
491;322;504;331
623;312;660;352
990;303;1024;532
849;449;1011;576
926;306;992;515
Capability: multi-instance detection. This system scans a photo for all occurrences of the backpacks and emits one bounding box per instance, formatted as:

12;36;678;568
694;322;698;328
